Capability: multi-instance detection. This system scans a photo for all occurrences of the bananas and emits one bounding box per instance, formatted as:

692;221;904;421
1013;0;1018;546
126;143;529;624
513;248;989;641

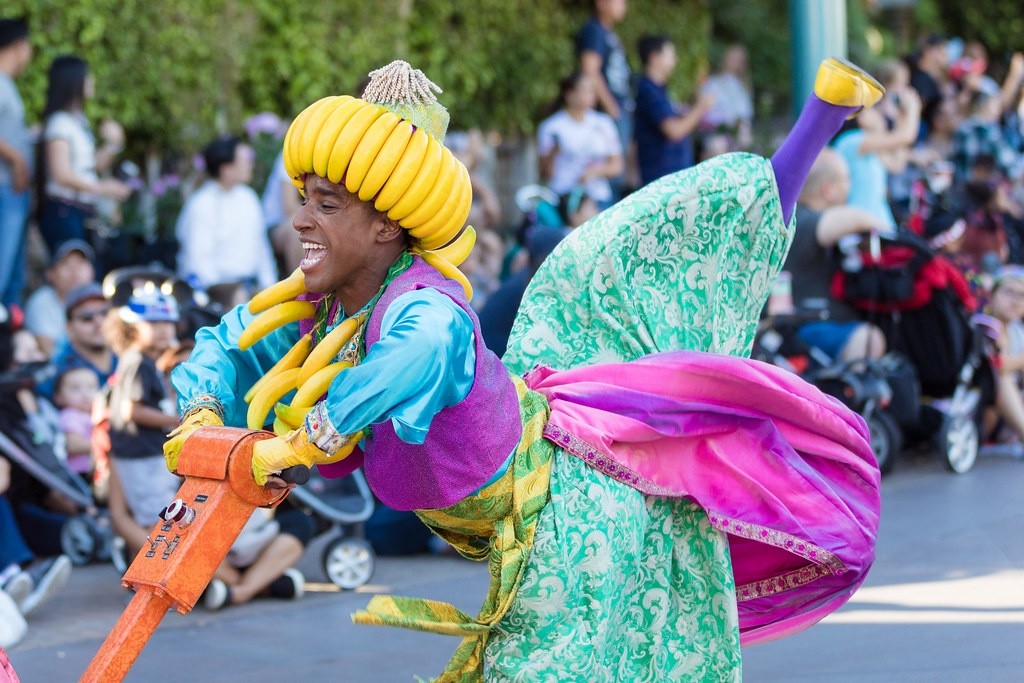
413;226;476;302
240;266;363;464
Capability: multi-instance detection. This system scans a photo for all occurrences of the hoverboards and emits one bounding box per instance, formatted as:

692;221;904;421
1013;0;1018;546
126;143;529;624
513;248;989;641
79;425;310;683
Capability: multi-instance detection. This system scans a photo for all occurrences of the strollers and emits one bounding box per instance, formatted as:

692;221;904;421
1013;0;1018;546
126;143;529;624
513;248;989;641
0;268;376;590
745;228;1007;478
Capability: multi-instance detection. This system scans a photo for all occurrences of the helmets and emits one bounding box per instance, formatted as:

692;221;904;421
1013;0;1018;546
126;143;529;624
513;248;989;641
127;289;181;322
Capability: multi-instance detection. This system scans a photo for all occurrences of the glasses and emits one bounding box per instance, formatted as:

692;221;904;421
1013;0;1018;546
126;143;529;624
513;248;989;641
71;305;113;323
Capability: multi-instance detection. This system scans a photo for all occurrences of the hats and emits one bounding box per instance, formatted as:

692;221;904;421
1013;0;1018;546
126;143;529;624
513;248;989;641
50;238;98;264
63;282;105;312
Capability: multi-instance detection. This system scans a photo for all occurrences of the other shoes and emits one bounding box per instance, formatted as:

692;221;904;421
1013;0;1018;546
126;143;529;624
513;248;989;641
7;554;72;620
200;578;234;610
110;535;136;575
267;568;307;602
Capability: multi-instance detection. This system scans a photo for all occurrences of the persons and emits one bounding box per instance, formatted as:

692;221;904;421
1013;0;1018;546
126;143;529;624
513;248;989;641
0;18;315;619
164;55;886;683
785;35;1024;449
449;0;753;363
